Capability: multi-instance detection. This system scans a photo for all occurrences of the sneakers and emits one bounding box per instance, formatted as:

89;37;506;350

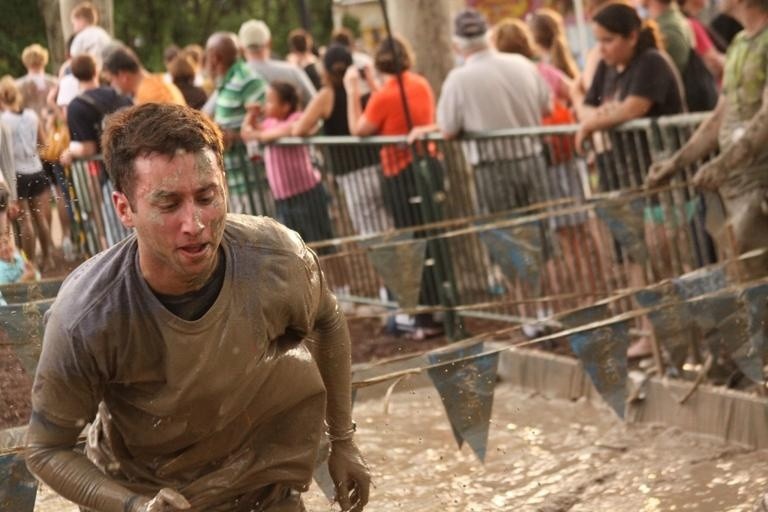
37;237;83;275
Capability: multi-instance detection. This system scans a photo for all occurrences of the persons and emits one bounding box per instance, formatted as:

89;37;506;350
1;1;766;356
22;103;371;512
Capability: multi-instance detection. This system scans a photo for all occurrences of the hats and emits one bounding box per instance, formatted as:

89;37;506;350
238;19;271;47
451;10;485;38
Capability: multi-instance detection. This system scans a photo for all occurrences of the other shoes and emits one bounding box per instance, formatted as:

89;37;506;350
411;327;443;343
524;318;566;339
626;336;658;357
341;298;357;318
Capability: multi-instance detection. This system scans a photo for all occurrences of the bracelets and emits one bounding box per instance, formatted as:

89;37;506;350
324;419;356;440
